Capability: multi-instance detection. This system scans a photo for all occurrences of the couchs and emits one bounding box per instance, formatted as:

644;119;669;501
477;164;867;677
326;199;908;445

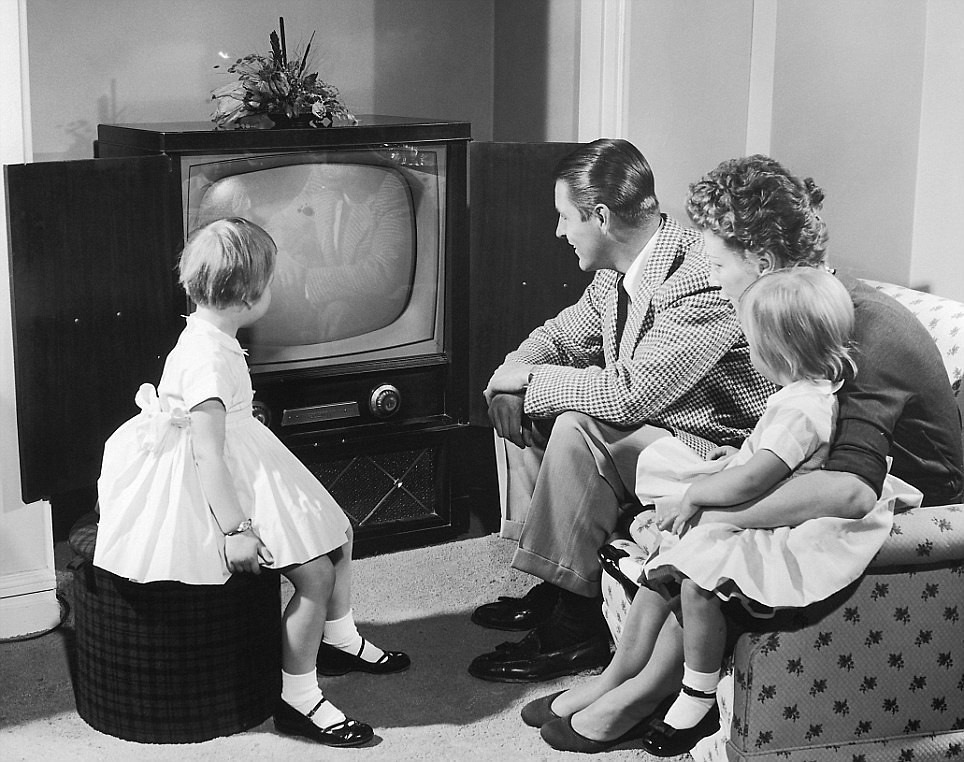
596;277;964;762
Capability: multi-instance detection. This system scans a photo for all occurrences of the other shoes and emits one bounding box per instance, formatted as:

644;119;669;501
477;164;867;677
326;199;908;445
521;688;569;728
541;695;676;754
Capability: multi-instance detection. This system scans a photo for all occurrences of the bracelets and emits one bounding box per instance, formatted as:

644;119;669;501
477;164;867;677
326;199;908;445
223;519;252;537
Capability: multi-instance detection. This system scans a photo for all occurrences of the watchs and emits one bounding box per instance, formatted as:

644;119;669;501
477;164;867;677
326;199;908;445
528;364;541;383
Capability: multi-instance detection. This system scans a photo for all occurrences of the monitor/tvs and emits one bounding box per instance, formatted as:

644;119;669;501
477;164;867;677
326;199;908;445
164;139;474;445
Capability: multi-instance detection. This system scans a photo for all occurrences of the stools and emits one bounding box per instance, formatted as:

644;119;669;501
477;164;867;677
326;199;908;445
66;509;281;744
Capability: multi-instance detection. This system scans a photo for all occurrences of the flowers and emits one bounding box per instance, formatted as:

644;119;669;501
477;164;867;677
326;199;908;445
209;15;361;132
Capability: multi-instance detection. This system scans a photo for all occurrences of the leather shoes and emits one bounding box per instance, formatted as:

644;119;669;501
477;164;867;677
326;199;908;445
469;624;614;681
642;684;721;756
274;693;374;747
317;638;411;675
472;584;590;631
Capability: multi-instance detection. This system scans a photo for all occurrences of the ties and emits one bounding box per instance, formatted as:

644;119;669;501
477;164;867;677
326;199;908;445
338;199;351;263
615;276;628;359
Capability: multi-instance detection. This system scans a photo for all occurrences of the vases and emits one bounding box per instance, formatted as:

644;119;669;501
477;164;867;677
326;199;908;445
270;106;323;128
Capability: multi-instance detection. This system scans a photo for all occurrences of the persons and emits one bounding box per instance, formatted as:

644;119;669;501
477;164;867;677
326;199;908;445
201;161;413;345
157;215;413;746
521;155;964;757
466;132;716;682
